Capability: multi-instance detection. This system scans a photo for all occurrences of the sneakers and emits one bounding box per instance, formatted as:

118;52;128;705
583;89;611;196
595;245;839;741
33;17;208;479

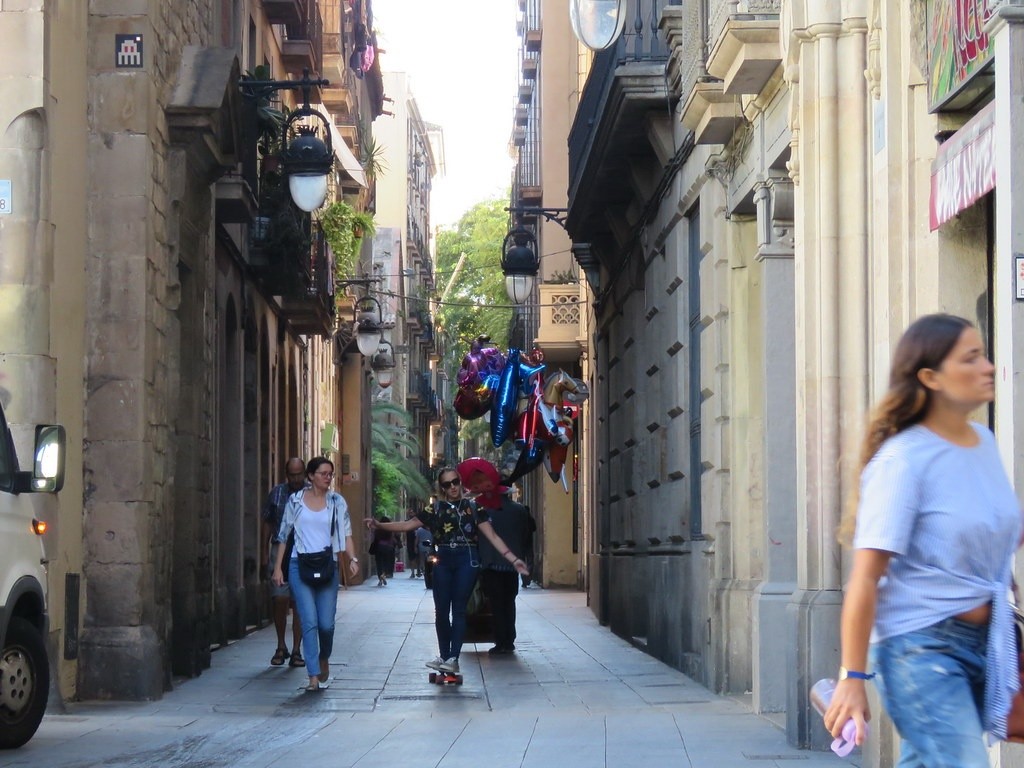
439;655;459;672
426;657;445;670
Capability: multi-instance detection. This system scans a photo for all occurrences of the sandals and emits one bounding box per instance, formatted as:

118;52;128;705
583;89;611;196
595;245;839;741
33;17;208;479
271;646;290;666
290;653;305;667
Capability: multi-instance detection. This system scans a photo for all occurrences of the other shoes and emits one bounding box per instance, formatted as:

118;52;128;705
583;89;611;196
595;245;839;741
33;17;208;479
306;683;319;692
489;644;514;654
318;659;329;683
378;575;388;586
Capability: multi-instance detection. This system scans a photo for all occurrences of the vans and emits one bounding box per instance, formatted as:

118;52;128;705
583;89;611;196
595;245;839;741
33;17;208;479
0;403;66;749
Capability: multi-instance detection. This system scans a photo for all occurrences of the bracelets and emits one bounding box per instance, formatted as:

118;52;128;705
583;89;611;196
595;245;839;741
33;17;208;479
504;550;511;556
512;558;518;564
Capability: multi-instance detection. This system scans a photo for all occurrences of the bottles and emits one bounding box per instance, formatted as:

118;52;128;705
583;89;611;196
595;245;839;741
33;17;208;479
810;678;869;758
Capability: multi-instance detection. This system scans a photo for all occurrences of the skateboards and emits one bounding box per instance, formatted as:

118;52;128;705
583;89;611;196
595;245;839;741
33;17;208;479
429;670;463;685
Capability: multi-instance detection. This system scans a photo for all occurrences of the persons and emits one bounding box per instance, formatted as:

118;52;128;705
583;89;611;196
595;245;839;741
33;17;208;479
405;509;432;578
363;466;529;672
478;479;528;654
369;517;396;586
824;313;1024;768
271;457;359;691
263;458;311;668
520;506;537;588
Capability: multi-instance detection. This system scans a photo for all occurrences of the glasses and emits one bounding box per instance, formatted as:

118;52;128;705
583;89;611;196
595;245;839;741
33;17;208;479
313;472;335;479
442;478;460;489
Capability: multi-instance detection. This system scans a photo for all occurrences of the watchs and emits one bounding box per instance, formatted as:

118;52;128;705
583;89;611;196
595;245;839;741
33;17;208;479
350;557;358;563
839;667;875;681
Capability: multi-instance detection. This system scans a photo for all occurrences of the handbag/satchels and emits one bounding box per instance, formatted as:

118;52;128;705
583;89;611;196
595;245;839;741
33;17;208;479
296;546;331;584
369;542;375;555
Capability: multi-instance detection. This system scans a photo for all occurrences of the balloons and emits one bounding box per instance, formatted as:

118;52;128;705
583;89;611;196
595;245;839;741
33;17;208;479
452;334;579;483
457;456;499;493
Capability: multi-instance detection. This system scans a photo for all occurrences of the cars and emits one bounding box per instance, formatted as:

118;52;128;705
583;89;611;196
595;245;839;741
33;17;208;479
422;539;439;589
